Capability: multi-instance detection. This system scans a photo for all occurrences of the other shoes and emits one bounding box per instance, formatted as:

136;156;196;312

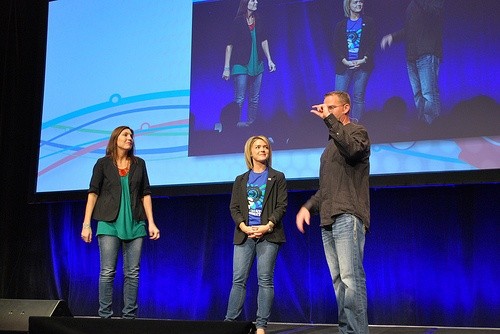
255;325;267;334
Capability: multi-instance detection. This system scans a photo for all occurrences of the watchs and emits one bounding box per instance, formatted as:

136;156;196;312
266;226;273;233
364;55;368;64
296;91;370;334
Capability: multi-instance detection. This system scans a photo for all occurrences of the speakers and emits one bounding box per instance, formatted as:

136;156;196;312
0;299;73;334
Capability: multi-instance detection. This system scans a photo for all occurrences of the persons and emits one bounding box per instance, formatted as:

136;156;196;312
332;0;376;123
188;96;500;156
81;126;160;318
224;136;287;334
219;0;276;123
380;0;443;124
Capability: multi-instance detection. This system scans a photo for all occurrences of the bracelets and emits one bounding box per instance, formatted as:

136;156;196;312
224;67;230;72
83;223;91;229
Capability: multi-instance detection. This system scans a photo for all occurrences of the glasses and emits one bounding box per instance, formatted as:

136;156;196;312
328;105;344;111
